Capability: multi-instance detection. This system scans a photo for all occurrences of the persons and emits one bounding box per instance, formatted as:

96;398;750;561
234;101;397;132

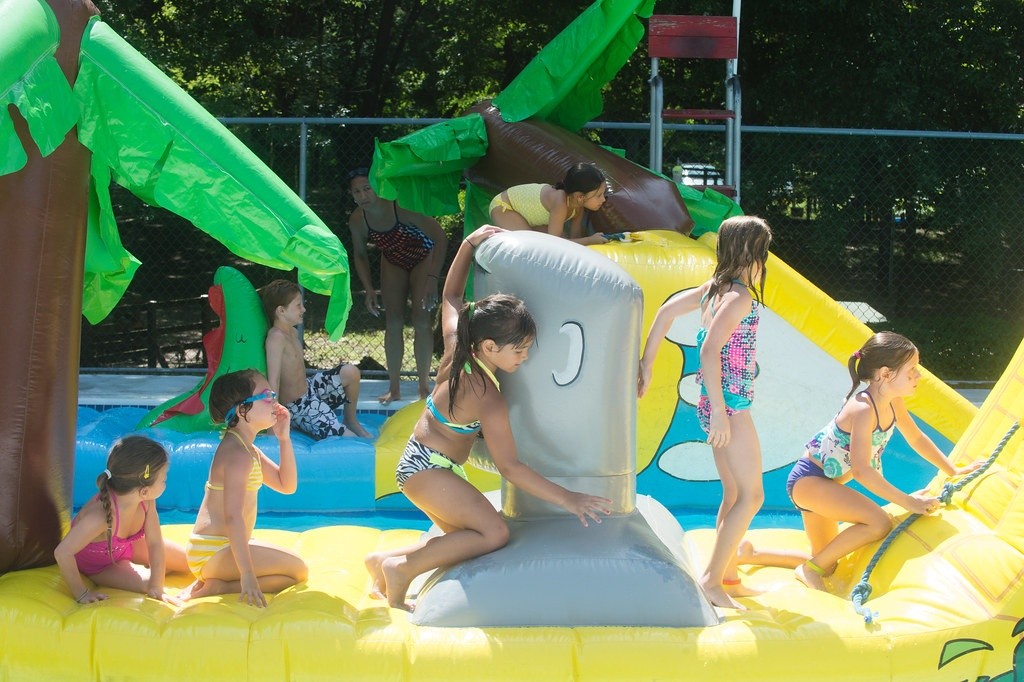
737;330;991;593
177;368;309;608
638;214;774;611
489;162;610;245
261;279;376;439
348;166;448;403
53;435;191;607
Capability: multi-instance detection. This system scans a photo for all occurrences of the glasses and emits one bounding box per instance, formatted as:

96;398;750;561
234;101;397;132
241;390;276;404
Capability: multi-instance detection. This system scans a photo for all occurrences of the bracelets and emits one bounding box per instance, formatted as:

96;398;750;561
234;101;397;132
427;274;439;279
76;588;90;602
363;222;612;613
465;237;476;249
